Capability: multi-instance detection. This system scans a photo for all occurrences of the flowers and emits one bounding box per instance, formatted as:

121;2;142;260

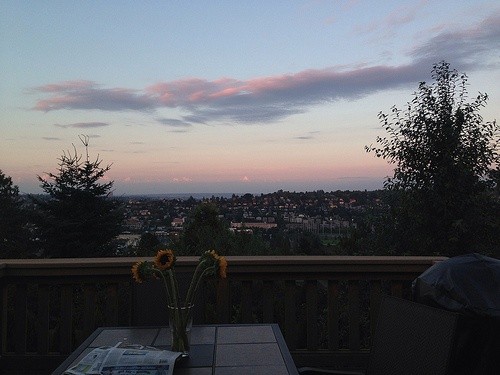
131;248;228;307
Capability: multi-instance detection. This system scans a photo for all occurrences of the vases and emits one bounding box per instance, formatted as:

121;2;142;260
167;302;195;366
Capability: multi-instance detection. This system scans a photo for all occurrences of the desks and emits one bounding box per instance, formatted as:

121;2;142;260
50;322;299;375
